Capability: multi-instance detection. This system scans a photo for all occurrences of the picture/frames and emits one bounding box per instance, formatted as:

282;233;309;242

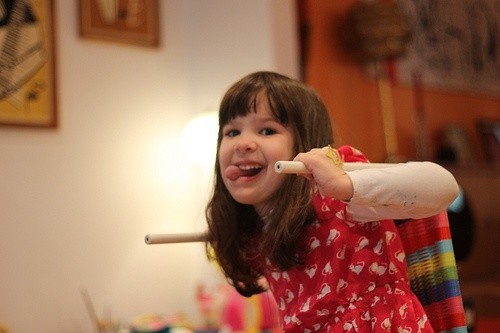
80;0;160;48
0;0;58;129
